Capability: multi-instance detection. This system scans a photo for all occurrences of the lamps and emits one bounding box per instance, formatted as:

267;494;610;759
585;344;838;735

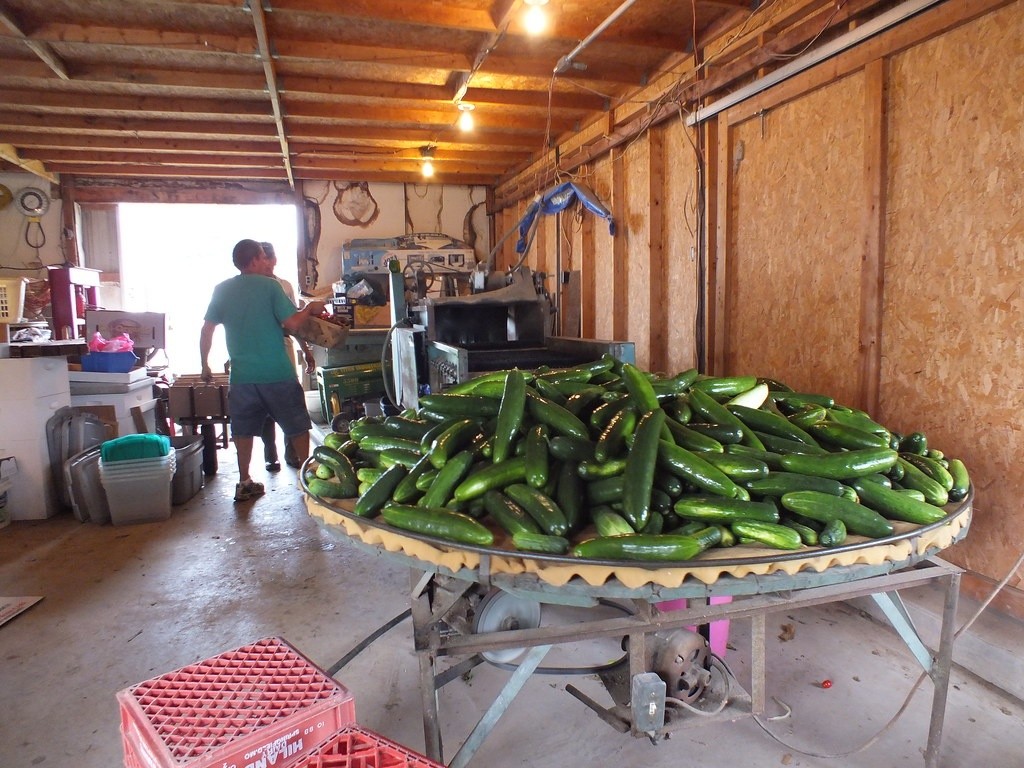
419;144;437;180
456;103;477;131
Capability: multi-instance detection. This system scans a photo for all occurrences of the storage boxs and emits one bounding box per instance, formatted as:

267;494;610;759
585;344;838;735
315;360;397;424
43;406;209;527
116;633;452;766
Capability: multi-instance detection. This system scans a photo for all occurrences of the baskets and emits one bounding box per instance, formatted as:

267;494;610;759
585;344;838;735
115;634;356;768
280;721;452;768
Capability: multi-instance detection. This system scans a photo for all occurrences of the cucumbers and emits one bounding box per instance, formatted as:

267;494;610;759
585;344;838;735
305;352;969;563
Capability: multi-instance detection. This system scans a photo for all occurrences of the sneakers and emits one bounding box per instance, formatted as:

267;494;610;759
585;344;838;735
264;446;281;471
233;483;265;501
